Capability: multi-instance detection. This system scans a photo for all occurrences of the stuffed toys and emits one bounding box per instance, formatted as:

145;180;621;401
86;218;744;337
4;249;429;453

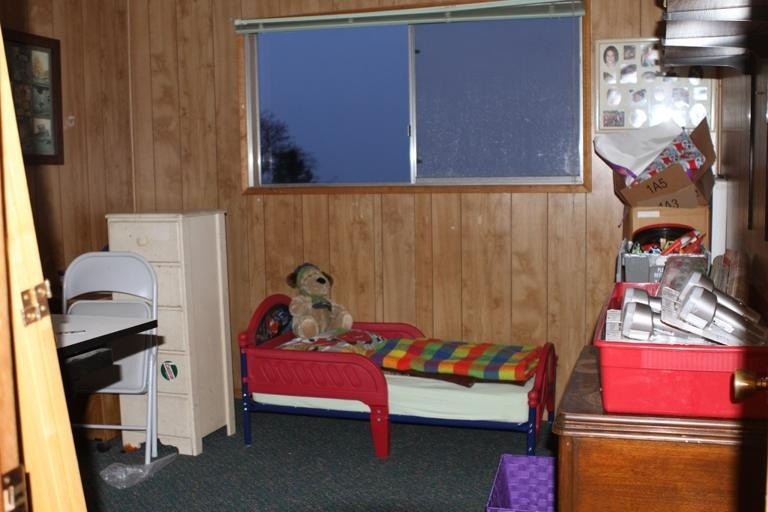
287;262;353;339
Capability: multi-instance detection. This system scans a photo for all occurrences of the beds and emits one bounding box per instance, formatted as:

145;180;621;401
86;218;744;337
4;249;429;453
239;294;556;458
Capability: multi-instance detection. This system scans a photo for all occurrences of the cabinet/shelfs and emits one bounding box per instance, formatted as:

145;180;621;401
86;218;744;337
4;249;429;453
105;209;237;457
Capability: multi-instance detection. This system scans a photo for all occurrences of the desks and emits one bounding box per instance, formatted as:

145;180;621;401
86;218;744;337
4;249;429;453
50;312;159;394
554;344;768;511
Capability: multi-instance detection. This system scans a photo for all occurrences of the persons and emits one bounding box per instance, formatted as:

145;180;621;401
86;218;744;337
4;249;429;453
604;47;618;69
603;111;624;126
632;91;645;102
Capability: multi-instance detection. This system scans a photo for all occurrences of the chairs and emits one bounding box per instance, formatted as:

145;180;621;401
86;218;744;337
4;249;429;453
62;251;158;466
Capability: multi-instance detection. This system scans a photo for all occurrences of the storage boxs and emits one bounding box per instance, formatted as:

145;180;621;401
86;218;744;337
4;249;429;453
614;117;716;249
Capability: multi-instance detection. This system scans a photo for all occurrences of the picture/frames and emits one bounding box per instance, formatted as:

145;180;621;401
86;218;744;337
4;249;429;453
2;28;65;167
589;36;717;135
